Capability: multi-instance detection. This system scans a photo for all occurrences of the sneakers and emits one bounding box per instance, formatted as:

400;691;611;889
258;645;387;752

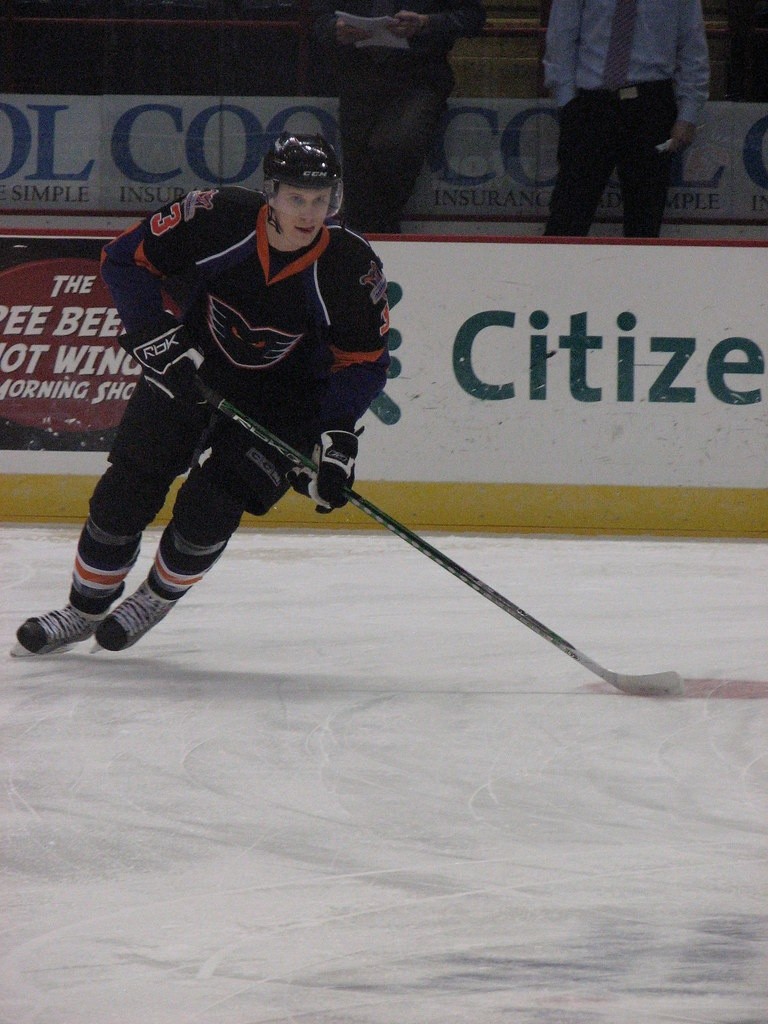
90;578;178;654
11;603;111;657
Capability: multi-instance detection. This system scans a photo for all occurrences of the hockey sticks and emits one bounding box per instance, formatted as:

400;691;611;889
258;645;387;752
182;373;688;701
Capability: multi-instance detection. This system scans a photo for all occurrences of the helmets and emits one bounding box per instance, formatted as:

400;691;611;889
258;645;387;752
262;133;341;189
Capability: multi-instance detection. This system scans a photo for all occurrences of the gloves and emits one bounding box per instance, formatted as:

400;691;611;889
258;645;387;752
118;311;210;404
288;429;358;514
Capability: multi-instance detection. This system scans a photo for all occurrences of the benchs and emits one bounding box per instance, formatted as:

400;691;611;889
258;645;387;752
0;19;312;95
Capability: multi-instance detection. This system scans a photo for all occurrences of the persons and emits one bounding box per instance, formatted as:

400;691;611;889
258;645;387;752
300;0;487;235
543;0;710;238
10;132;390;654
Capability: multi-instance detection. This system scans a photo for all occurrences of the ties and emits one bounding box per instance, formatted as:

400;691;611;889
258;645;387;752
603;0;638;92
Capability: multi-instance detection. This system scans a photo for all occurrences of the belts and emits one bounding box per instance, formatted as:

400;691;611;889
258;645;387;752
582;82;672;101
